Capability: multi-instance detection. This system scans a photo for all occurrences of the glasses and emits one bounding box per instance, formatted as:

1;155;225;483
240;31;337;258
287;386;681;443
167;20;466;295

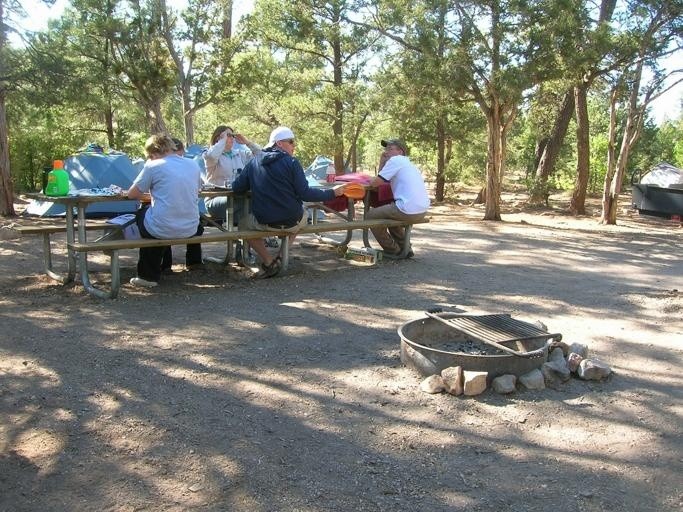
280;140;294;145
226;133;238;137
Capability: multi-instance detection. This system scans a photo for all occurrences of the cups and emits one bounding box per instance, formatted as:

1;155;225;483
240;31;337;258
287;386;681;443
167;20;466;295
233;168;242;186
224;178;232;188
41;166;52;195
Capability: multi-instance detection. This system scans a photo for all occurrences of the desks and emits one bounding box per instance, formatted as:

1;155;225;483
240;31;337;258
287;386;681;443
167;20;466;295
26;176;393;205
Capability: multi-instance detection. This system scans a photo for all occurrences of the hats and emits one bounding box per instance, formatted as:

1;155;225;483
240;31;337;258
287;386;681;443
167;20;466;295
381;137;408;153
263;127;294;151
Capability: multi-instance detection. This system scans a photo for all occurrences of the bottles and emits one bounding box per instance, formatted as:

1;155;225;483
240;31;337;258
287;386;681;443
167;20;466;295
325;162;338;185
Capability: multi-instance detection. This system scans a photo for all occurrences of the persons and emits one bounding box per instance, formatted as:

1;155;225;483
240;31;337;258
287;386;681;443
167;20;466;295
201;126;279;266
129;131;200;287
171;138;204;270
365;139;431;259
231;126;345;280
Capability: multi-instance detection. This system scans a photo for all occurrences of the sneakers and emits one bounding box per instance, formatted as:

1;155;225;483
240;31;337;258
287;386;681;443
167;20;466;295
128;278;159;290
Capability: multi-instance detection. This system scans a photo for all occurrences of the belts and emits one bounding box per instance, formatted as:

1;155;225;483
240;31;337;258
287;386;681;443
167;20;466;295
268;216;302;230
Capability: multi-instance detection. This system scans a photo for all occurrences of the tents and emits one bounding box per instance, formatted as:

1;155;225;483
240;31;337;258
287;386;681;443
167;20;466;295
183;143;207;215
22;148;145;217
304;154;352;186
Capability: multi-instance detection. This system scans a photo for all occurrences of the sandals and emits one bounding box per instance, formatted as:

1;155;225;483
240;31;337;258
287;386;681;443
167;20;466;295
252;260;279;281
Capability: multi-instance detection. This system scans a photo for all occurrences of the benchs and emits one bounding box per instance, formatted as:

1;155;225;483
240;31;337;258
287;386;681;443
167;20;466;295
67;218;430;298
13;202;328;284
